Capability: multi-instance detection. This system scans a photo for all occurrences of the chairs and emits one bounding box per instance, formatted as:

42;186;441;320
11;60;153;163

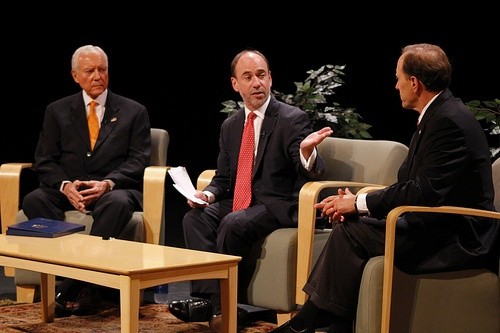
354;157;500;333
196;136;409;327
0;128;171;303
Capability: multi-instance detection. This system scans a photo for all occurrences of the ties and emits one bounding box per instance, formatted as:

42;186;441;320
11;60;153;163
87;102;100;150
232;112;257;212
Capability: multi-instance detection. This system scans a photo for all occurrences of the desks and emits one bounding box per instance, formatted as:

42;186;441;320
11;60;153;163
0;232;242;333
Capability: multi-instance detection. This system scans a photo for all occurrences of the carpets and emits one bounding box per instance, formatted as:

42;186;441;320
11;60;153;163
0;297;277;333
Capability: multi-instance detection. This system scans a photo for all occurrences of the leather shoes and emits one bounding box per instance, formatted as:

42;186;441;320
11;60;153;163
168;298;211;322
55;292;66;309
72;297;96;313
269;321;315;333
209;306;244;331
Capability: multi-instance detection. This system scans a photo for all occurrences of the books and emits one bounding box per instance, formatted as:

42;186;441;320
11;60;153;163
5;218;86;238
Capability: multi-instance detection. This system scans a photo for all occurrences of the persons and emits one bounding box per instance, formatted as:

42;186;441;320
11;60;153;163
167;50;334;333
22;45;152;318
268;44;500;333
